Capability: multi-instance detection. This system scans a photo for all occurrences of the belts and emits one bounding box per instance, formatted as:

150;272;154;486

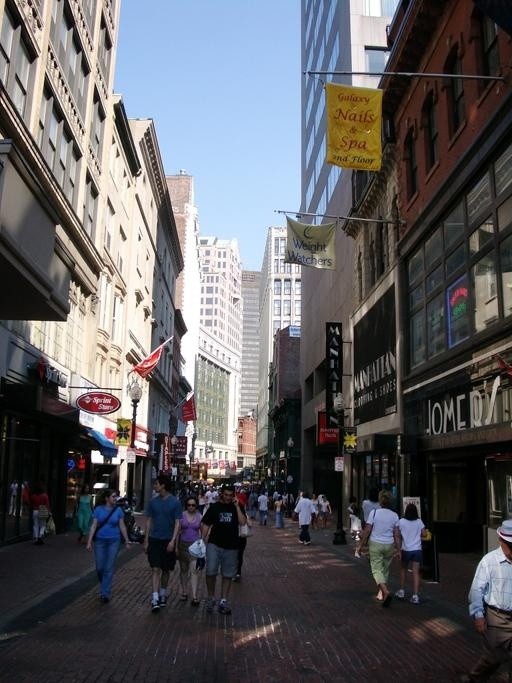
485;602;512;614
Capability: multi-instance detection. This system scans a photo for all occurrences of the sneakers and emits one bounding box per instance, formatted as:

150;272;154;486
181;595;231;614
395;589;405;598
152;596;167;609
410;595;420;604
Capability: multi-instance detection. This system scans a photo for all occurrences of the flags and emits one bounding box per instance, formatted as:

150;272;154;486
283;214;337;271
181;394;196;423
134;345;163;378
342;426;358;455
323;80;384;171
114;418;132;446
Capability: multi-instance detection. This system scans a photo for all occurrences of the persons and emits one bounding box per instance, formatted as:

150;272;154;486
356;489;402;604
349;489;379;542
74;485;102;545
31;483;50;545
7;479;31;520
235;484;302;529
144;475;252;617
395;504;427;604
86;488;132;603
458;518;512;683
311;493;332;529
293;491;316;545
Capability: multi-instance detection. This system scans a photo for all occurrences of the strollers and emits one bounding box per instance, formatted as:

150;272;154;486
113;498;145;546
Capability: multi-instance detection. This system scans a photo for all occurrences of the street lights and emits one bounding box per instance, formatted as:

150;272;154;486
284;436;295;517
170;434;178;482
271;452;276;493
196;460;201;481
242;466;256;496
122;380;142;524
258;461;263;494
189;451;193;485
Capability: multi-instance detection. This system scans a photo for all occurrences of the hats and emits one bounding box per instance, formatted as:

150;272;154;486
497;519;512;542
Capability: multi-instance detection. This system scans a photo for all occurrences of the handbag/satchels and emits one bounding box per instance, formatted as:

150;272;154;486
238;523;253;538
